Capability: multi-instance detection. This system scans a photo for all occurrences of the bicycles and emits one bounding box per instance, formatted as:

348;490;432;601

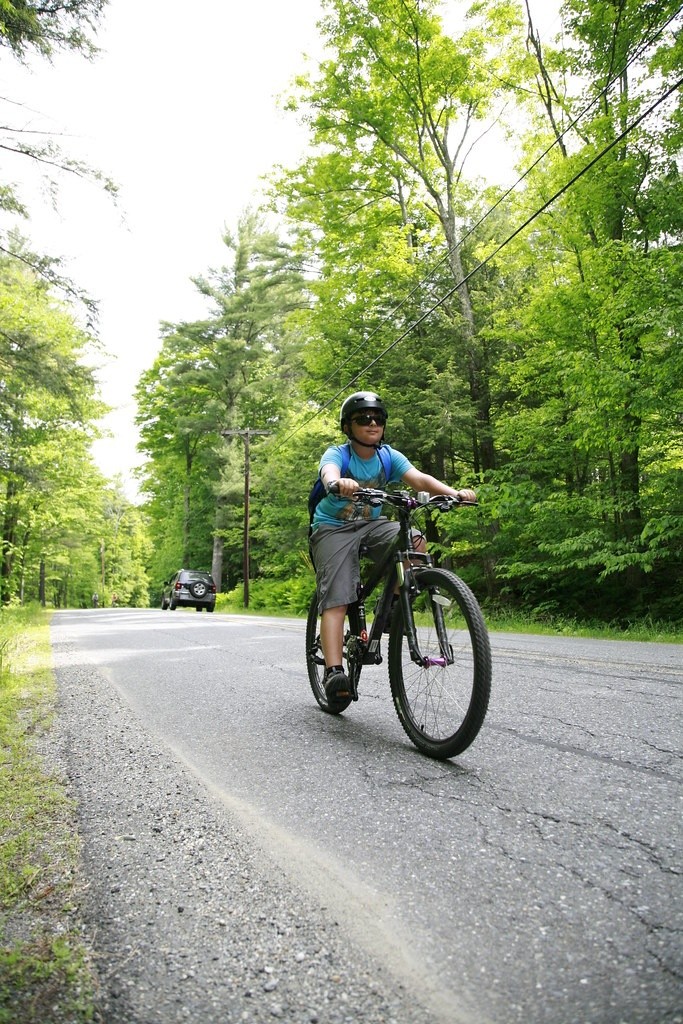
305;483;494;761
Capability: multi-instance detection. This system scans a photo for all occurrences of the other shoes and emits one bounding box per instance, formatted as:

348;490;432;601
372;598;397;634
321;667;352;712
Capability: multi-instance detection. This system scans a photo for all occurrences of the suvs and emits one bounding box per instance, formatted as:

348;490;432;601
161;568;217;613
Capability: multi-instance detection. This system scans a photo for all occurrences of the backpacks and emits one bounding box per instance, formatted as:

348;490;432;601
308;445;393;519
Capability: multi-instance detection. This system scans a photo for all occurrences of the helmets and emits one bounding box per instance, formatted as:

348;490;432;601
340;391;388;420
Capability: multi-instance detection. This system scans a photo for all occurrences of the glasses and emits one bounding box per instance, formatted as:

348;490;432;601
350;415;387;426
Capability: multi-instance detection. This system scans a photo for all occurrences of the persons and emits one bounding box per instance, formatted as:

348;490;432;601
112;593;117;604
93;592;98;603
308;392;477;704
